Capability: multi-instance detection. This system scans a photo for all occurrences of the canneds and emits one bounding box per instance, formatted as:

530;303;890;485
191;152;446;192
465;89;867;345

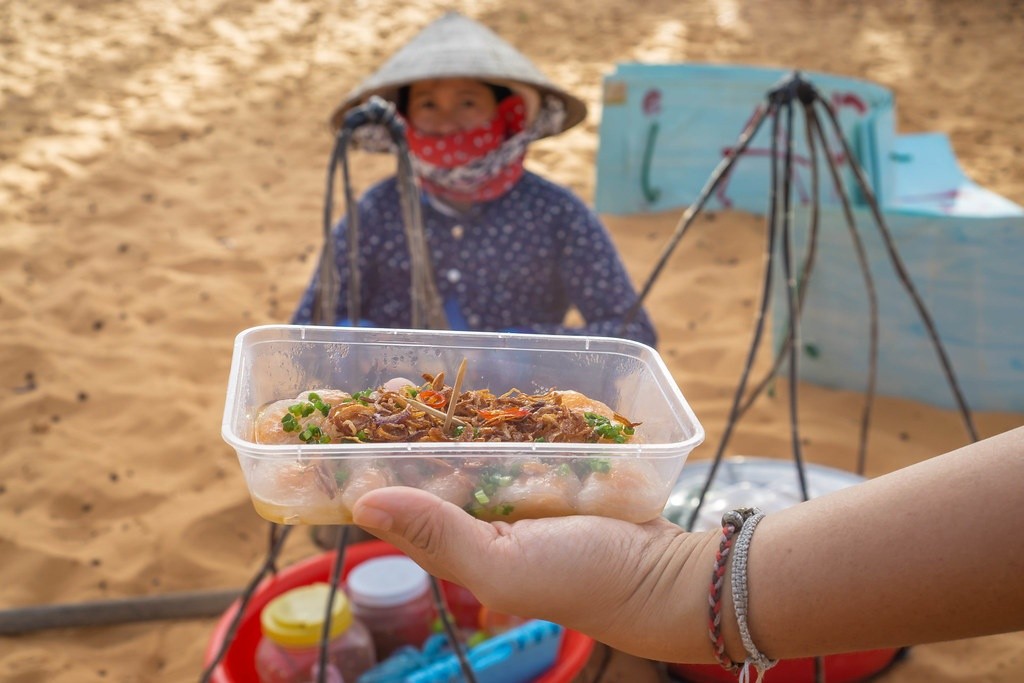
253;553;529;683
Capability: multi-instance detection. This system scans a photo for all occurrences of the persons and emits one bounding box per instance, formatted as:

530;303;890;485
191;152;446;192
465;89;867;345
352;425;1024;664
287;12;657;549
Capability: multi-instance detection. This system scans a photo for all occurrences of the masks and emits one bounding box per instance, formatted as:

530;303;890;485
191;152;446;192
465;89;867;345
395;94;526;204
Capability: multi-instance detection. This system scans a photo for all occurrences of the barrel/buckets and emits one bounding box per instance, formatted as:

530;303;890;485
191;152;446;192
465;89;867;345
207;536;599;683
641;456;905;683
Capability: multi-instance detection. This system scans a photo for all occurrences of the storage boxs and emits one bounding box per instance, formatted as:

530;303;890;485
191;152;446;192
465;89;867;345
661;459;912;683
216;324;708;531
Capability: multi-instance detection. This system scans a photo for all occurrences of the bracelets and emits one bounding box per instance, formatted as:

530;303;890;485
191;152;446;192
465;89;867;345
706;506;779;683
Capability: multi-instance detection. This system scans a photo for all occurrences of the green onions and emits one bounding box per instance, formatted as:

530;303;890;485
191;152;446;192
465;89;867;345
282;387;636;519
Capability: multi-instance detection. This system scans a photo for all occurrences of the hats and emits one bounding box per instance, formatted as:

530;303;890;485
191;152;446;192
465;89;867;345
327;10;586;141
